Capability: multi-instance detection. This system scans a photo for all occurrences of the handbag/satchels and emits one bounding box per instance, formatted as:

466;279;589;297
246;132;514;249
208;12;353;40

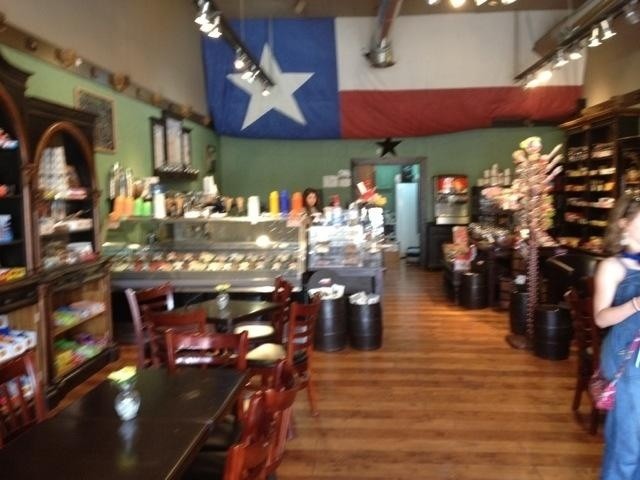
587;368;617;410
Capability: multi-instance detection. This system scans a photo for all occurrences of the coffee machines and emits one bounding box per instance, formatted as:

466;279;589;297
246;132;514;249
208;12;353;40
432;174;471;227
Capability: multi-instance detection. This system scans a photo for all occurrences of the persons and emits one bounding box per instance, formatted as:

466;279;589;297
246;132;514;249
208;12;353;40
592;189;640;479
303;188;321;213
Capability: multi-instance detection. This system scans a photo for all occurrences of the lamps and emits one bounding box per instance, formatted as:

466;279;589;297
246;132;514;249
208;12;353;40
194;0;275;97
514;0;640;91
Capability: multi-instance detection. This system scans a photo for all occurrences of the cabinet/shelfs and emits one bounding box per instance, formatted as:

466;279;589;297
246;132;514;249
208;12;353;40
0;274;47;427
308;212;383;293
588;106;616;254
429;225;469;270
33;94;101;274
101;215;304;295
617;87;640;254
0;52;33;282
47;256;119;409
472;185;515;227
557;116;589;252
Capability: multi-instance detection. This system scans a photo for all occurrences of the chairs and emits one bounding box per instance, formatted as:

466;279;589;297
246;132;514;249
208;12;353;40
224;391;270;480
0;350;48;450
125;277;321;418
267;359;291;475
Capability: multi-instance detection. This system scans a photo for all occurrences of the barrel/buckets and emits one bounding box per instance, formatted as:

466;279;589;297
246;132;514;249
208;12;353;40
510;291;530;334
348;294;382;351
308;283;349;353
532;302;573;361
458;270;488;310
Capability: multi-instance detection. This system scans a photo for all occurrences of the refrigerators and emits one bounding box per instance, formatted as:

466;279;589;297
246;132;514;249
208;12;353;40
395;182;421;258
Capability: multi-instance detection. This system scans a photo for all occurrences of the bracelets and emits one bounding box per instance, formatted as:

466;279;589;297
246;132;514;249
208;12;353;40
632;296;639;314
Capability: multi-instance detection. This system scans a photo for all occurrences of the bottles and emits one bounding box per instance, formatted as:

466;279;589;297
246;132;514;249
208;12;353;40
144;198;151;215
247;196;258;218
106;364;141;420
477;163;510;186
215;283;230;310
151;184;167;218
280;189;289;213
291;192;303;210
269;191;279;214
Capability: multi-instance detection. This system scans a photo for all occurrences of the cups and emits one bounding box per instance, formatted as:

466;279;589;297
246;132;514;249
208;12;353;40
39;145;67;190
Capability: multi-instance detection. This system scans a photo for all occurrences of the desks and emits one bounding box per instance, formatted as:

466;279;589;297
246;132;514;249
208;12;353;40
0;363;247;480
